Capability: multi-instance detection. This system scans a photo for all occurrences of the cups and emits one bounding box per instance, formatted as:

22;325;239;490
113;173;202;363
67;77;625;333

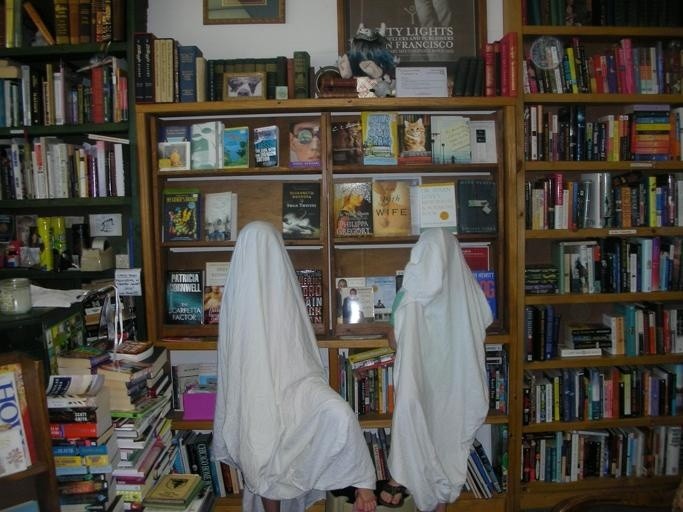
1;278;32;316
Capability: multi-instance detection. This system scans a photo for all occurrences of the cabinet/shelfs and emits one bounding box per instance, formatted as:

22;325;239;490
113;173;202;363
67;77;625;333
503;0;683;512
327;94;519;512
137;98;330;511
0;0;154;288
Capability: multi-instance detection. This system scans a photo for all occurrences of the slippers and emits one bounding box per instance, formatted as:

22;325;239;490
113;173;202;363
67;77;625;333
378;484;405;508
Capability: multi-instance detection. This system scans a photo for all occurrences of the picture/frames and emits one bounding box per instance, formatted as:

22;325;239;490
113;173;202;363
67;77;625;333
222;69;267;100
337;0;487;77
203;0;284;25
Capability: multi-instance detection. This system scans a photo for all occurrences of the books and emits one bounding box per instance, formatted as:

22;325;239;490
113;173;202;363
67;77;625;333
0;0;680;512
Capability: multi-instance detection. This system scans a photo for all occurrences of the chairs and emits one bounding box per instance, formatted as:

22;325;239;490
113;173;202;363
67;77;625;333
549;477;683;511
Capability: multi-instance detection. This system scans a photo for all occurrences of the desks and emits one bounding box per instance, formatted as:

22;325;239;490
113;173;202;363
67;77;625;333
0;274;115;383
